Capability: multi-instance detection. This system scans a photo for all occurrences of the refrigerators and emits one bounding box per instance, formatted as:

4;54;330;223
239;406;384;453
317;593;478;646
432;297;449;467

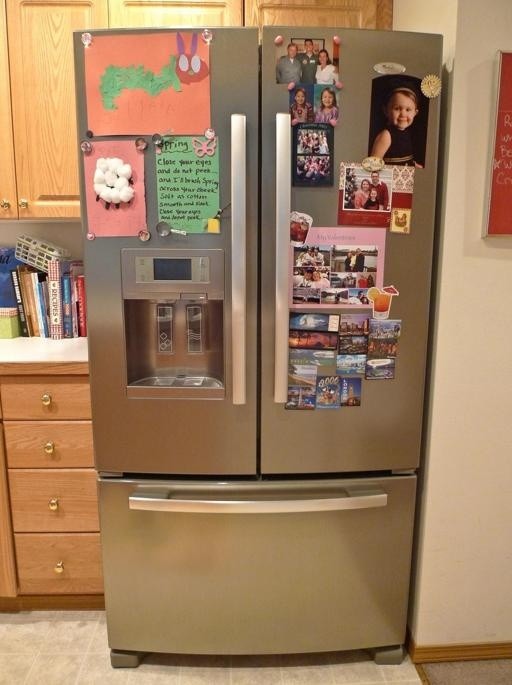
73;26;445;669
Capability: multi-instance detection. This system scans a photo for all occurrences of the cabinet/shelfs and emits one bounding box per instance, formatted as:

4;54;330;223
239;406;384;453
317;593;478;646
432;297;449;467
3;368;106;614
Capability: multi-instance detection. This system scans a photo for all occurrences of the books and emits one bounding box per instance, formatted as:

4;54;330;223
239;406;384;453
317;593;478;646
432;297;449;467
8;253;89;344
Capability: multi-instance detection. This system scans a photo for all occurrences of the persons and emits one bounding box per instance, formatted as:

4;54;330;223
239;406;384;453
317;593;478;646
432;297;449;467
363;188;380;210
276;43;302;83
292;245;374;305
367;170;389;211
294;127;331;179
314;48;339;86
315;88;340;124
277;39;338;85
290;85;314;123
371;87;424;169
355;178;370;211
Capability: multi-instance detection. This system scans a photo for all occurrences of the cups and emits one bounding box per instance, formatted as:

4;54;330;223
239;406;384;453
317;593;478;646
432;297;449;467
289;212;313;249
373;293;392;320
